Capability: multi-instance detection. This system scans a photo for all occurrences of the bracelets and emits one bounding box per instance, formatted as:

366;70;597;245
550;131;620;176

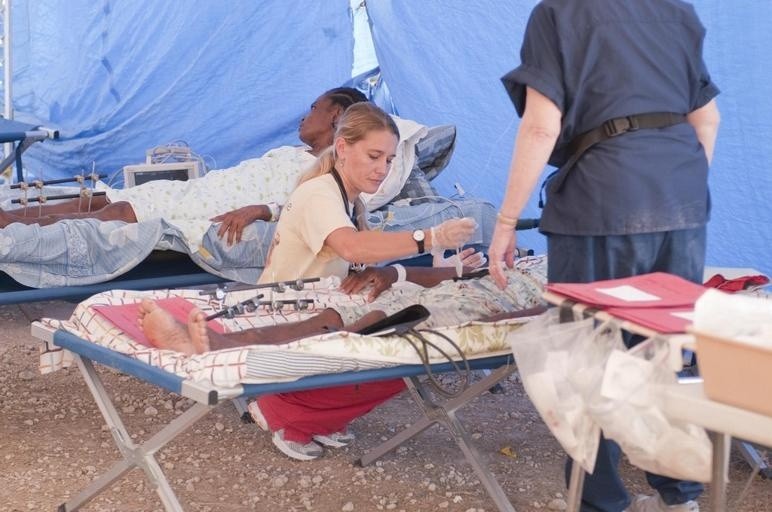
389;264;406;282
496;213;518;226
267;202;280;222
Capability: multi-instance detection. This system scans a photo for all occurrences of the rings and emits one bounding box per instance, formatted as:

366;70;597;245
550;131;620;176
491;264;496;268
355;269;361;274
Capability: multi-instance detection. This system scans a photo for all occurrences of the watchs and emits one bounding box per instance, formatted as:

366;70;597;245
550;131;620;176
412;228;426;254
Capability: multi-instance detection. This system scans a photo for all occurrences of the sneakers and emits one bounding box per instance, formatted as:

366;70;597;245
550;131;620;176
249;401;323;460
634;489;698;511
307;426;356;449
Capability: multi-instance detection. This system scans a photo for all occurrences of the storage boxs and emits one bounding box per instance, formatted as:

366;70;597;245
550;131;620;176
684;325;771;416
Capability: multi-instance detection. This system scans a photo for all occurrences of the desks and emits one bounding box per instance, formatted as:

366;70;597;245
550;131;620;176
566;352;772;512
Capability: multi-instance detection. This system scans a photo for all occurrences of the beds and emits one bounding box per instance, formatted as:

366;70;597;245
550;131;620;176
2;116;62;184
2;188;545;310
26;239;767;512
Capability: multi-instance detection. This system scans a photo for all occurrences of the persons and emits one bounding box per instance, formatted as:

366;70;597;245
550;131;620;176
247;101;478;462
486;1;719;511
135;251;549;356
0;86;368;247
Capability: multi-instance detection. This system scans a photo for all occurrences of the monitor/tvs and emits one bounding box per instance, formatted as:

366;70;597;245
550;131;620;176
123;162;199;187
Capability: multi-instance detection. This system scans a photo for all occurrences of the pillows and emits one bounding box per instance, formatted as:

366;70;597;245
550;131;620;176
360;116;428;211
